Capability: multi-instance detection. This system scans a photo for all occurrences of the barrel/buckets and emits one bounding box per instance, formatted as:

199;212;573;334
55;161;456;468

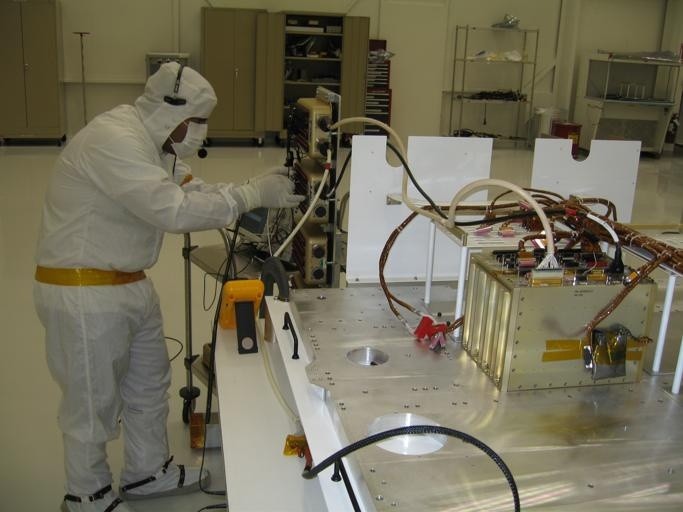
552;120;582;158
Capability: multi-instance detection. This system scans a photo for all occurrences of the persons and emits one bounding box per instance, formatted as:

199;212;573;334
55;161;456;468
32;58;309;511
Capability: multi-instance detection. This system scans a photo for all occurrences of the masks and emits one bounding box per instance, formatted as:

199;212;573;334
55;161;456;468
169;120;209;159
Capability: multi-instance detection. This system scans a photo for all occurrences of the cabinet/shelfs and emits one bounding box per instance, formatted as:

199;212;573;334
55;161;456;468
0;0;66;147
198;7;391;147
570;52;682;158
447;25;540;150
